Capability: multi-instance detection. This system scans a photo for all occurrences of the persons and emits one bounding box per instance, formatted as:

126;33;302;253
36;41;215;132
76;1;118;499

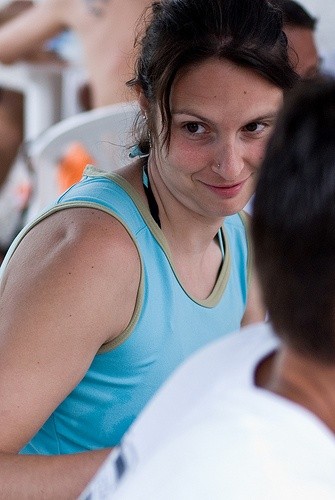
1;0;156;111
77;72;333;496
267;0;324;82
1;1;303;497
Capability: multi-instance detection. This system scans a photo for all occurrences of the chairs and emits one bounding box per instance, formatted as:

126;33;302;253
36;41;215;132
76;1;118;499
3;31;153;247
